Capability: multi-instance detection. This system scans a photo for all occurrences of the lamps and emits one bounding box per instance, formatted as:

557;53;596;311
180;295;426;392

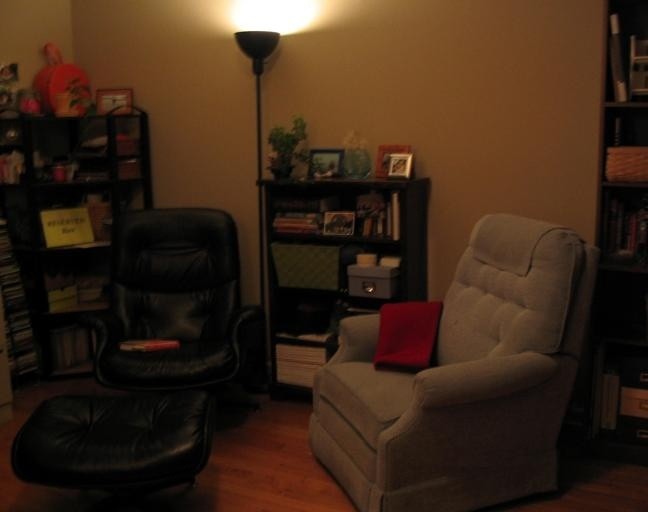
237;29;280;392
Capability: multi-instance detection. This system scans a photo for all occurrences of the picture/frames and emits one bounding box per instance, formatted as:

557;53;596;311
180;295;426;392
376;143;413;180
310;146;346;178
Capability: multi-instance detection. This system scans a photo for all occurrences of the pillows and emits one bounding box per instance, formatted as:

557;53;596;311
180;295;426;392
369;292;443;367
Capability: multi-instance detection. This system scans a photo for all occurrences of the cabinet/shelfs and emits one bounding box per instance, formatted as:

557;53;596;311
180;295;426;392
253;170;432;403
0;106;150;386
594;2;646;455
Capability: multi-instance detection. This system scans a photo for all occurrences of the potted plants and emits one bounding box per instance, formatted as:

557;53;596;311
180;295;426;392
265;120;303;177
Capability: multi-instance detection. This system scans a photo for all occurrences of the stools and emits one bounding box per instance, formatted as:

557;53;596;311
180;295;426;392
12;389;216;507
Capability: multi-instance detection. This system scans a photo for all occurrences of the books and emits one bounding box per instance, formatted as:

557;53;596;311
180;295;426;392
272;194;325;238
50;322;97;371
602;199;648;255
362;192;401;242
0;219;38;378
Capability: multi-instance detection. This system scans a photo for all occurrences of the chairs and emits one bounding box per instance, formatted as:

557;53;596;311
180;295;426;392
308;211;587;511
96;204;270;395
